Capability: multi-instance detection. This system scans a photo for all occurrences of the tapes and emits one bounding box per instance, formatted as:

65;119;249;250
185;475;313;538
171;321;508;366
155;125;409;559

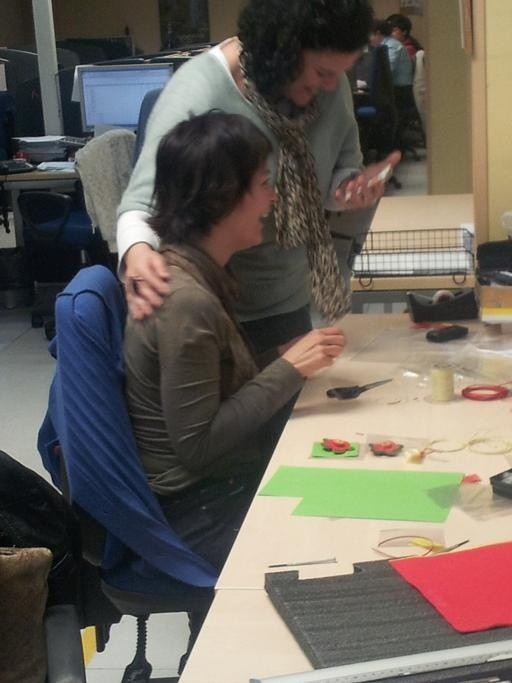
431;290;456;304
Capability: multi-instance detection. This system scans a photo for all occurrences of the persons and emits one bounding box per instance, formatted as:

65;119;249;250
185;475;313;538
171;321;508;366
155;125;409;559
116;1;402;429
346;12;427;183
121;108;347;568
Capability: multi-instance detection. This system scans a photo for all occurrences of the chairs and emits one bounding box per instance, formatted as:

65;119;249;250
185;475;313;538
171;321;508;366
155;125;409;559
18;129;140;334
38;265;233;681
354;45;427;189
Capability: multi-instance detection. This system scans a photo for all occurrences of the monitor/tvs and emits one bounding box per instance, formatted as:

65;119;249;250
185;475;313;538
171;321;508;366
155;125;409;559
76;63;175;132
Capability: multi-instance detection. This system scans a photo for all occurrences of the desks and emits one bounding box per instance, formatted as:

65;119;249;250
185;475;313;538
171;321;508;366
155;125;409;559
178;313;511;682
348;194;478;313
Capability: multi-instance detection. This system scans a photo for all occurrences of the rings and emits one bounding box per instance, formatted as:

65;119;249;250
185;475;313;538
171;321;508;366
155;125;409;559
128;275;144;295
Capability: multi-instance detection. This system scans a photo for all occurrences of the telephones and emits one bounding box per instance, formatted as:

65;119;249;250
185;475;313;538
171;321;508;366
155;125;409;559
0;158;37;176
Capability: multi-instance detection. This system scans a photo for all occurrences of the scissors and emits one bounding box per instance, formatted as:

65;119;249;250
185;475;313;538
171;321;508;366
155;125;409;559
325;377;393;401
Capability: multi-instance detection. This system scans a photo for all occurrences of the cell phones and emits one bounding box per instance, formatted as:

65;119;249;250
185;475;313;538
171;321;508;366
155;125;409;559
344;163;393;202
426;325;468;342
